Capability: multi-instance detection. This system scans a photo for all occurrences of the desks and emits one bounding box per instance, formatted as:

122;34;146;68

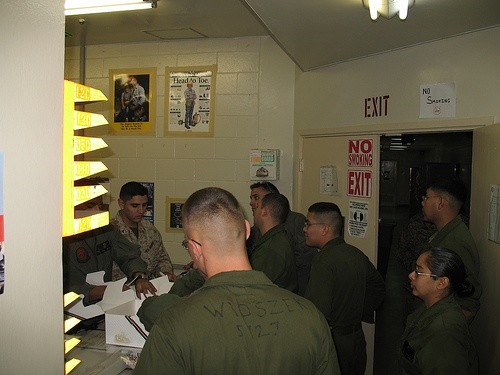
64;319;141;375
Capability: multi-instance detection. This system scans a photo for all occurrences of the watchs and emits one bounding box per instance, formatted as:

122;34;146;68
135;274;148;279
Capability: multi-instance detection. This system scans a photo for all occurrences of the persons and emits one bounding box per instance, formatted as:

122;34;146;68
397;246;479;375
138;181;318;332
63;179;158;329
135;186;341;375
184;80;197;129
302;201;385;375
115;76;145;122
108;181;177;282
406;172;485;325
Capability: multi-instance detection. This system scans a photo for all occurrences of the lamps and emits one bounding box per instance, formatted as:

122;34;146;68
64;0;158;15
361;0;415;23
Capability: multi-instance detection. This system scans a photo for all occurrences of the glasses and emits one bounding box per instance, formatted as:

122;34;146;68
181;238;202;250
422;195;449;202
304;217;326;226
412;269;441;279
